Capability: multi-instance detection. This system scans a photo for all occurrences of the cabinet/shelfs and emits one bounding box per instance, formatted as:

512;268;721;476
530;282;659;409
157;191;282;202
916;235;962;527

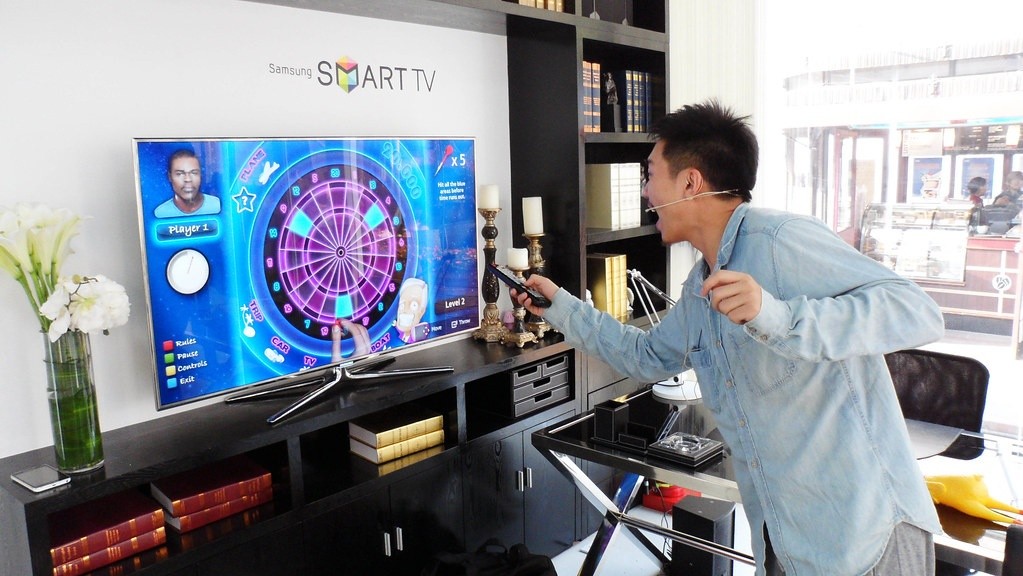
0;0;671;576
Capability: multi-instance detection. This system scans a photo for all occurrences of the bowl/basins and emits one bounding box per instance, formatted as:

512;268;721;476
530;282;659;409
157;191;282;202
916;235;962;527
977;226;988;234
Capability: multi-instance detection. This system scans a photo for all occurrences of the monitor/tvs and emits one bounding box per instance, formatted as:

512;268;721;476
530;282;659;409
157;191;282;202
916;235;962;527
133;135;481;423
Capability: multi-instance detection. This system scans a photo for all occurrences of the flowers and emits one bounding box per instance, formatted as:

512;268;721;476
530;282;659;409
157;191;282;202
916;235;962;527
0;217;131;470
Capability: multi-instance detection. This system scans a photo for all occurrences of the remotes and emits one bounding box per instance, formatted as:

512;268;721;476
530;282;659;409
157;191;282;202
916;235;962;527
489;263;553;308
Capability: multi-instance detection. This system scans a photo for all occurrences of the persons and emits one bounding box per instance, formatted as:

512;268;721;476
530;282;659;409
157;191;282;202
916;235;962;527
964;177;987;225
155;150;220;217
992;171;1023;206
509;104;946;576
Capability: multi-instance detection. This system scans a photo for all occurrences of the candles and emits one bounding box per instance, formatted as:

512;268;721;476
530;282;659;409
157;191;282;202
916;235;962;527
522;197;543;235
477;185;500;210
506;248;528;269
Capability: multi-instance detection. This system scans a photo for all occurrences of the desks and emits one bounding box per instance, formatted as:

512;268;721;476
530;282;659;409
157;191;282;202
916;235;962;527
531;386;1023;576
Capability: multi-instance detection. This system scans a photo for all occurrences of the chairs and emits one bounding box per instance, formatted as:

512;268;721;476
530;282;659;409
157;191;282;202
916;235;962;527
883;349;990;433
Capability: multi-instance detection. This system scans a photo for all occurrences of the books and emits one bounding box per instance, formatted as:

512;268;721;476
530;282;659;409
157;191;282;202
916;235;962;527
29;457;272;576
356;444;445;476
583;61;600;132
586;253;627;324
348;404;445;465
513;0;565;12
614;70;653;132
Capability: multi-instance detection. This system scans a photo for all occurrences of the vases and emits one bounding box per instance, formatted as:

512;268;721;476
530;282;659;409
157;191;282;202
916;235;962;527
40;330;104;476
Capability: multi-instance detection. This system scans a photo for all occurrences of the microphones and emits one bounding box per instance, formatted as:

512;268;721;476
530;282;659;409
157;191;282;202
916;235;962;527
645;192;711;212
